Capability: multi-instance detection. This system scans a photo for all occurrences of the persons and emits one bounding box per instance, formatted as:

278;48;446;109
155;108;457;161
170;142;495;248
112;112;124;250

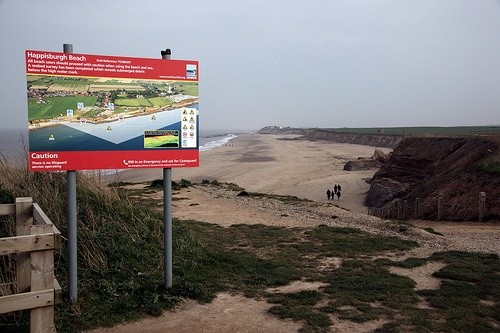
337;184;341;192
334;184;337;194
331;191;335;199
337;191;341;201
326;189;331;200
